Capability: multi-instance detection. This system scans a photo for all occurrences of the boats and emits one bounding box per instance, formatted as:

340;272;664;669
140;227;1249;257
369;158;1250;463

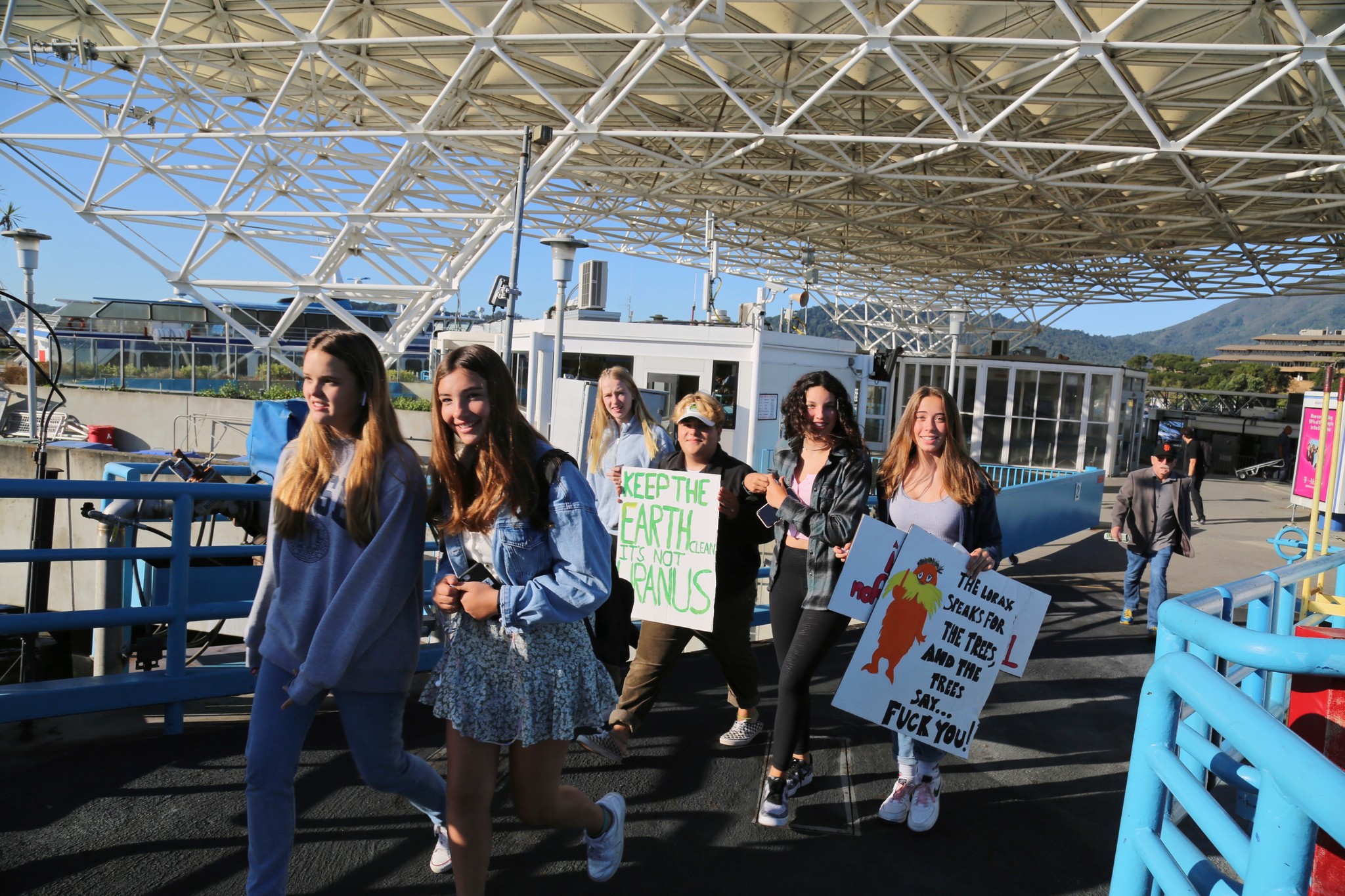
0;294;491;404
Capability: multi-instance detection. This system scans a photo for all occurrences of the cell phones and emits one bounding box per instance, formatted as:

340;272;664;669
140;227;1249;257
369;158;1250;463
457;562;502;621
755;486;802;529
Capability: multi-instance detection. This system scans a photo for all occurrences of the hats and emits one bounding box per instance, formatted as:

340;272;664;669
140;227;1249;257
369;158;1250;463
677;402;716;427
1152;443;1177;459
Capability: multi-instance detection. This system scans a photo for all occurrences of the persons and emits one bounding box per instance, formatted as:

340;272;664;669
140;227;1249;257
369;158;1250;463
758;369;874;828
575;393;762;766
1180;426;1206;526
833;387;1006;832
416;345;626;896
1110;444;1195;637
1263;425;1293;483
586;363;671;683
244;328;453;896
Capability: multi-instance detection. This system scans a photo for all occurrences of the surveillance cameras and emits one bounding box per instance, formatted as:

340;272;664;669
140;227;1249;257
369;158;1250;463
764;281;788;294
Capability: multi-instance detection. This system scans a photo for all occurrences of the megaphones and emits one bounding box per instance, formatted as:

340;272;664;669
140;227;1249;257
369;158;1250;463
789;291;809;307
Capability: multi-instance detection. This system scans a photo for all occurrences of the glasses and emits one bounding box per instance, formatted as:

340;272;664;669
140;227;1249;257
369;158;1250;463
1156;456;1174;463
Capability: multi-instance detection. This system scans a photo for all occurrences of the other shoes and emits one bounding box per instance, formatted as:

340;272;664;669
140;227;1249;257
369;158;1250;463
1193;518;1206;525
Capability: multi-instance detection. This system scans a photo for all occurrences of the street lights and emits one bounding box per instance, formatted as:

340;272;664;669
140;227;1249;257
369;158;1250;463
0;228;53;438
539;234;591;383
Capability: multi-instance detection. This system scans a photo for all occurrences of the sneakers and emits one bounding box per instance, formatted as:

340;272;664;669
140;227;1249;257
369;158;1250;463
878;778;915;823
1120;606;1134;625
584;791;626;882
430;823;451;874
786;752;814;793
907;774;942;831
758;776;789;826
1148;626;1157;642
576;727;624;763
719;720;762;745
